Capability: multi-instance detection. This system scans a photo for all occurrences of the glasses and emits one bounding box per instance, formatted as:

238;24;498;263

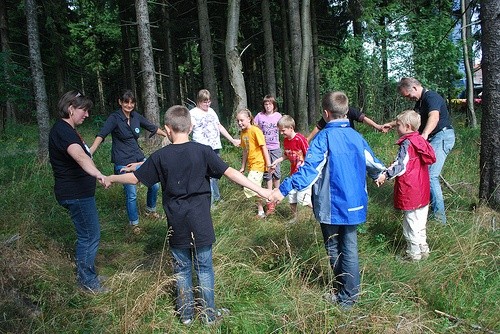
69;91;83;105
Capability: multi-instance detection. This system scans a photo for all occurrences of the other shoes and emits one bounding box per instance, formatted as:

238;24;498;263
144;210;163;220
288;217;297;224
266;202;277;215
202;309;230;326
254;214;267;220
82;275;110;294
132;225;140;235
395;253;429;266
211;199;224;210
324;290;338;301
182;317;194;324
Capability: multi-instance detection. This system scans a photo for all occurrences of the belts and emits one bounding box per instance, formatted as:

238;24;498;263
429;126;452;138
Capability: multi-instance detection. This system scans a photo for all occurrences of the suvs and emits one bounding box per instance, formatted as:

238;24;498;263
451;87;483;104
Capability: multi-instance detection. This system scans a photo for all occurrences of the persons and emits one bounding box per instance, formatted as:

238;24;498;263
88;91;165;234
49;91;109;294
305;106;388;143
188;89;239;203
374;110;436;264
251;95;282;190
268;91;386;310
104;104;273;324
270;115;311;225
237;107;270;220
380;77;455;228
